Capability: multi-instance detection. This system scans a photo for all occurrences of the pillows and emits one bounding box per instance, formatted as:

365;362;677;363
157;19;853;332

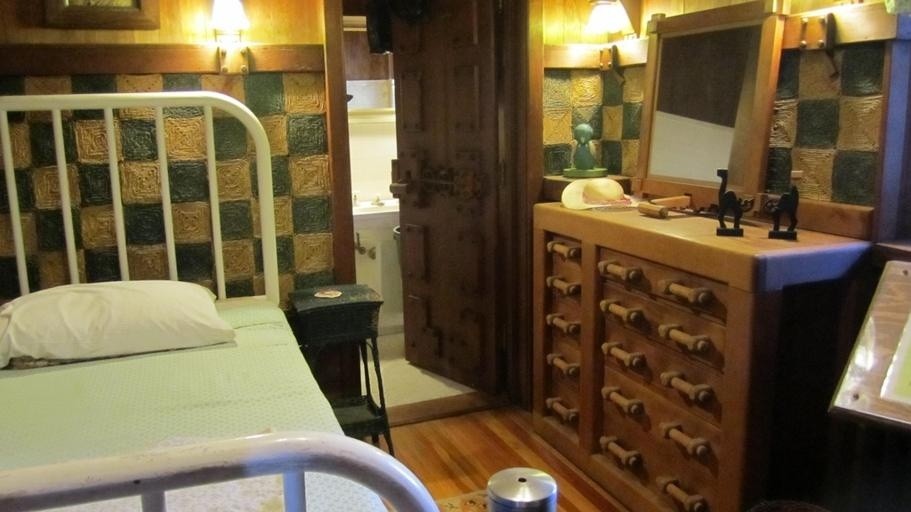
0;278;231;366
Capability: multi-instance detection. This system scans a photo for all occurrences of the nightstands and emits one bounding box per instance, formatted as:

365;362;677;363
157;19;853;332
287;284;396;460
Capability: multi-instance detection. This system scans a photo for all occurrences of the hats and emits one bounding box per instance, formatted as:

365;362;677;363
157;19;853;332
559;178;640;210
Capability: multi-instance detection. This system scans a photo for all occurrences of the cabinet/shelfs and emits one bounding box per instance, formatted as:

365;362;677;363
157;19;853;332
539;224;726;509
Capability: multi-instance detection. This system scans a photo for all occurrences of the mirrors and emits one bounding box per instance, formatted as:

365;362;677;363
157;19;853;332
632;5;786;223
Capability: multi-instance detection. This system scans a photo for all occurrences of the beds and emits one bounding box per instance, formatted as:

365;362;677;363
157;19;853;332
0;87;447;512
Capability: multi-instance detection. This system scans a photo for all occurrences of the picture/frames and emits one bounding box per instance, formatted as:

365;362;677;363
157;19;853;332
38;1;160;32
